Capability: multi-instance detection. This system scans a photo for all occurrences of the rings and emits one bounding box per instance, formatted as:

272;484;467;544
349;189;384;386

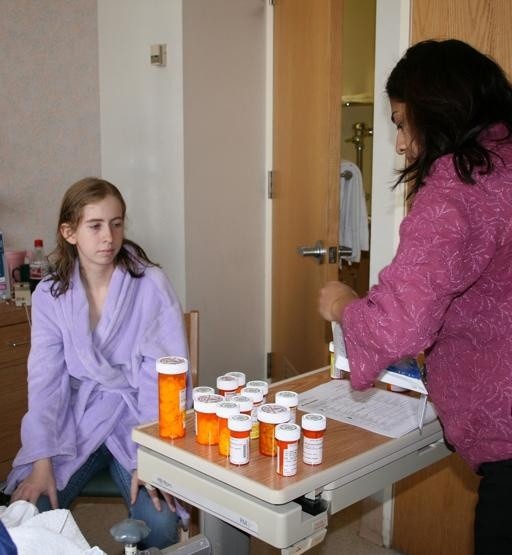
145;483;156;491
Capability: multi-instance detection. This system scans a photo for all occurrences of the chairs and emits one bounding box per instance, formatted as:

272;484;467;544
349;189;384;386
28;304;203;555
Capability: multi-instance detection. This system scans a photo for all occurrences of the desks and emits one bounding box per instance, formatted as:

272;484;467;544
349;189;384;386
130;343;462;555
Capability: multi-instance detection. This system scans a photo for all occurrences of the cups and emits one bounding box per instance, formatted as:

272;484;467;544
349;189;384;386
3;248;29;295
12;261;30;282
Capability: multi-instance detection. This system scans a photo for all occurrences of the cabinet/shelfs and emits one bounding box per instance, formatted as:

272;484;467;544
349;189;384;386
0;286;36;489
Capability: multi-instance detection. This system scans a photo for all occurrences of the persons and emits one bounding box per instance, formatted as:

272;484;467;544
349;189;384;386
319;37;511;555
1;176;191;551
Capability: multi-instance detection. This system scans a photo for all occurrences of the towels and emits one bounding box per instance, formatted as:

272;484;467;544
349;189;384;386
339;154;368;273
0;494;108;554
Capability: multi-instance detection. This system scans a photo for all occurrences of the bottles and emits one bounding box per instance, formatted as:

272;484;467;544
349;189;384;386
0;277;8;295
155;356;189;441
30;238;49;292
327;339;347;380
192;371;329;478
